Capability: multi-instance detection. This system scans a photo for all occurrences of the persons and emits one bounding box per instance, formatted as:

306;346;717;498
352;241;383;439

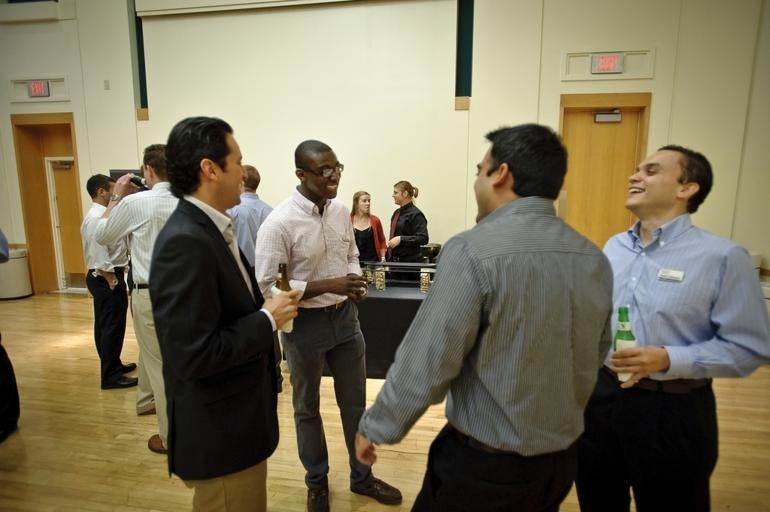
384;181;428;280
149;116;300;512
354;124;613;511
126;165;157;416
573;144;770;512
254;140;404;512
224;164;283;394
95;144;181;455
81;173;139;390
349;191;388;273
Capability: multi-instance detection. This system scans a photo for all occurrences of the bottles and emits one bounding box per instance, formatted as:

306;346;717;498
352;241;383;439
614;306;637;386
130;176;147;187
421;256;430;295
374;264;386;292
271;263;295;333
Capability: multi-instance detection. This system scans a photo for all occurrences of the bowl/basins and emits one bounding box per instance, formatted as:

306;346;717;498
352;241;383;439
420;246;441;258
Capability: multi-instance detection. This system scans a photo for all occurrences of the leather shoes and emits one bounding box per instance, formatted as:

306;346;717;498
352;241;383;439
122;363;137;373
148;434;168;453
101;377;138;389
307;485;329;512
351;477;402;505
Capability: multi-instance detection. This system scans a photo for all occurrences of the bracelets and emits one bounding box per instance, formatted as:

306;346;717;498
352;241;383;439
109;279;119;286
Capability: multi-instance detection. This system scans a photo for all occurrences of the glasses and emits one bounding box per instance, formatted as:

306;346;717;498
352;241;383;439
301;164;343;179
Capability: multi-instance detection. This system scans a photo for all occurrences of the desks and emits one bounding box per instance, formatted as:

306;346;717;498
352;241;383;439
357;280;433;379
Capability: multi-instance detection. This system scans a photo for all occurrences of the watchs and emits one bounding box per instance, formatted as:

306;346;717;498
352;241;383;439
110;194;121;201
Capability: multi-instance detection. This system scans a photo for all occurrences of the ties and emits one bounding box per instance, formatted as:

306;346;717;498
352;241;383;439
388;209;400;260
